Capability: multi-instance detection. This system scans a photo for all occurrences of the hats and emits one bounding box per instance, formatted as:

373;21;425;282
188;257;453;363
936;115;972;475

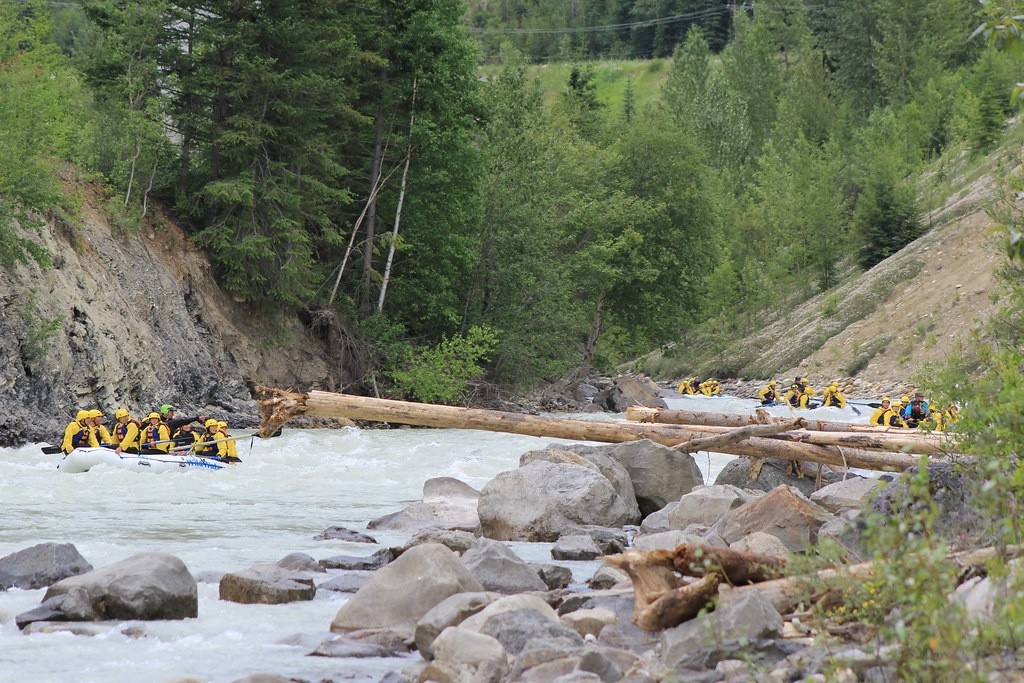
695;376;700;379
914;393;925;397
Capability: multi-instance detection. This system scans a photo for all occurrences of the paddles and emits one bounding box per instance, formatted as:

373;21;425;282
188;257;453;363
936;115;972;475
755;401;784;409
40;431;262;456
747;395;762;400
849;403;862;417
847;401;881;409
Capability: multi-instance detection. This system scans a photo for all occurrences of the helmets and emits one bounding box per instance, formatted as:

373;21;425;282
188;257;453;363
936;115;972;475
115;409;128;420
708;381;712;384
831;382;839;386
683;381;688;383
698;384;704;388
206;419;227;432
891;401;900;407
882;397;890;404
802;377;808;383
830;386;837;392
900;396;909;402
713;381;718;384
76;409;103;422
161;405;177;415
149;412;160;420
703;382;708;386
770;381;777;386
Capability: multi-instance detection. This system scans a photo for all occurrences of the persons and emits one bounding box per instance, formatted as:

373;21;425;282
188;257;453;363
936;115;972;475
823;382;846;409
870;392;959;431
61;405;238;461
785;377;814;409
679;375;720;396
758;381;782;405
